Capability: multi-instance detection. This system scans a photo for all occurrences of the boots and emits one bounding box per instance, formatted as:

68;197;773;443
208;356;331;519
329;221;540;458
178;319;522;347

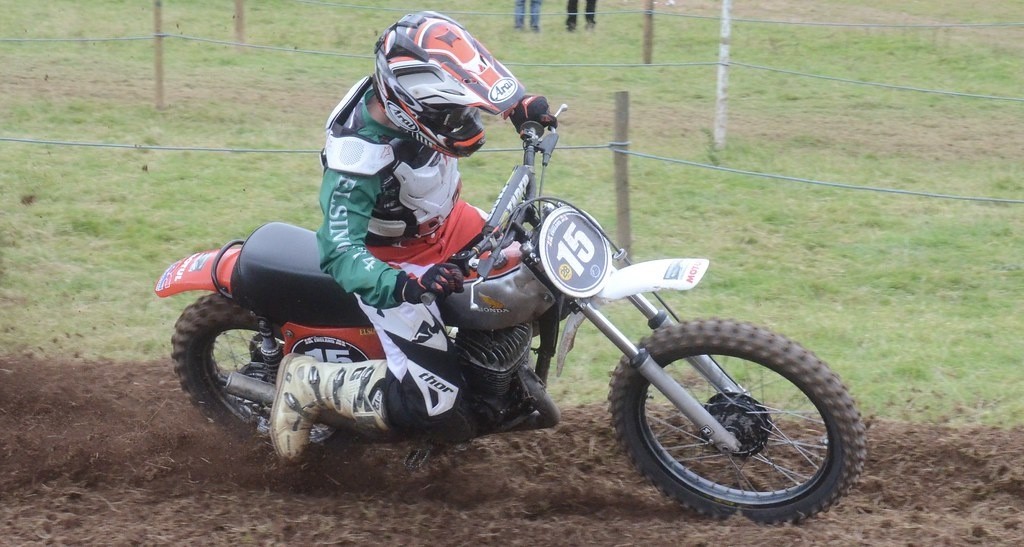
269;353;395;462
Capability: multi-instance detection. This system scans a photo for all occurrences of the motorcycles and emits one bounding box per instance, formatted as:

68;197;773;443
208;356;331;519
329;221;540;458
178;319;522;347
150;101;871;526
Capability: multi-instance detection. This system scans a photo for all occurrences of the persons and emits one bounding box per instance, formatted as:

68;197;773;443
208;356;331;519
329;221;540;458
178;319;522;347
269;11;557;460
513;0;596;32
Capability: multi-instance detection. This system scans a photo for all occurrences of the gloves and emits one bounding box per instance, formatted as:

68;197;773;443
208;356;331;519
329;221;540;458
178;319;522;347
509;93;557;143
393;263;465;307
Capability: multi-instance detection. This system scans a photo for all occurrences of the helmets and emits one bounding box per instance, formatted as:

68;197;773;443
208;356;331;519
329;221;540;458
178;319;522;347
373;11;525;157
